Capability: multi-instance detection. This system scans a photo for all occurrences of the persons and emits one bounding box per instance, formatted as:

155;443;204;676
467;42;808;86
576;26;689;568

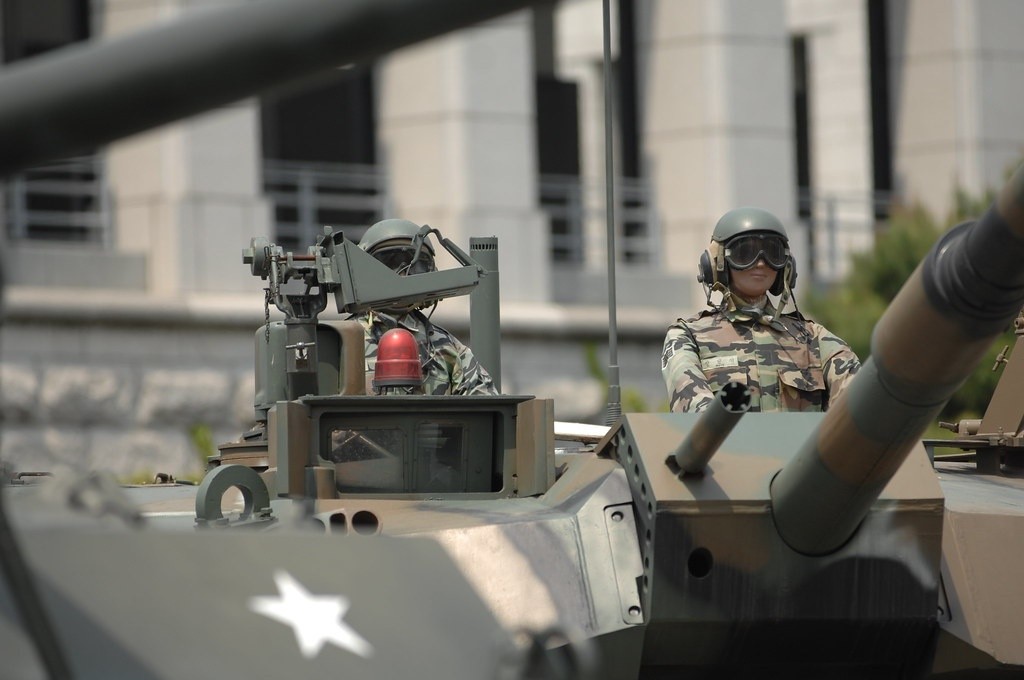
341;219;499;394
662;206;861;411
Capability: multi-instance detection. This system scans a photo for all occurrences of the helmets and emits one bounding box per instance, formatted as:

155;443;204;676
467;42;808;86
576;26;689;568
698;207;799;296
357;219;435;276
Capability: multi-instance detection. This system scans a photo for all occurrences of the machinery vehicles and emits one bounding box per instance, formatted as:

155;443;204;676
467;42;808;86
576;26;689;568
1;0;1024;680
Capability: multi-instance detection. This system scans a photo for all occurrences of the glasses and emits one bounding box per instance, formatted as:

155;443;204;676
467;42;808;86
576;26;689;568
723;234;791;270
370;247;434;276
